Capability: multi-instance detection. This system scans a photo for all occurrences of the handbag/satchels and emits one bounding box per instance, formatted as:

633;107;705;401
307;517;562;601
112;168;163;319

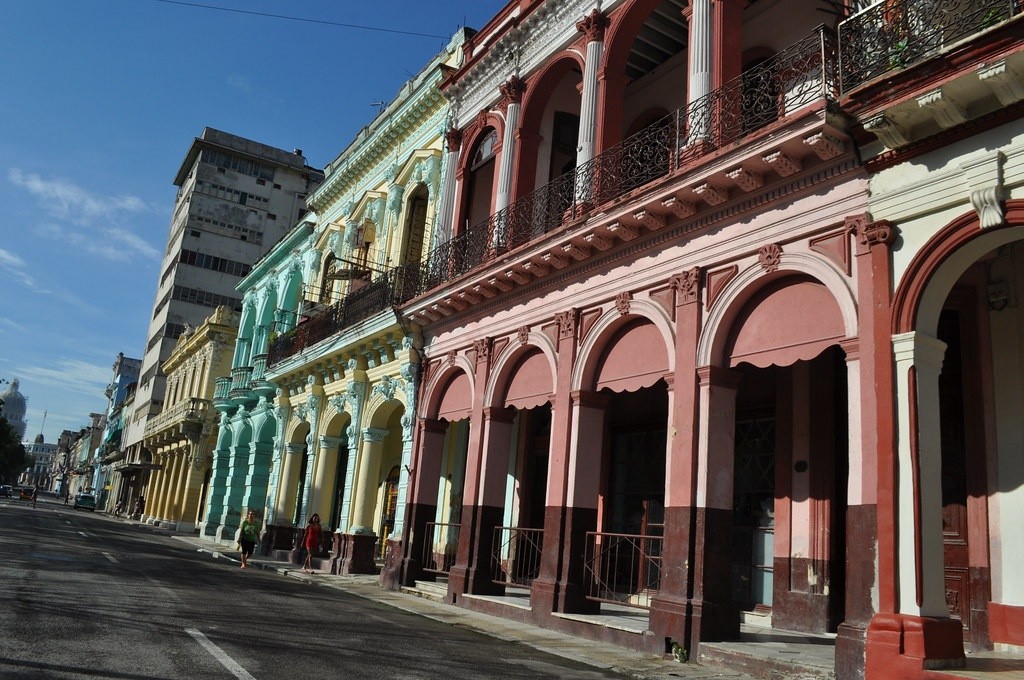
235;529;244;544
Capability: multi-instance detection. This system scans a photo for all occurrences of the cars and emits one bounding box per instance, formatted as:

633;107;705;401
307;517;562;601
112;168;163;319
19;488;33;500
73;492;97;513
0;485;13;498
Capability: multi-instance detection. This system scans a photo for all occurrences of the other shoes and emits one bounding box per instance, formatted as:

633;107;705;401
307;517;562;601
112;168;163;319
301;567;307;574
241;563;247;569
309;569;315;574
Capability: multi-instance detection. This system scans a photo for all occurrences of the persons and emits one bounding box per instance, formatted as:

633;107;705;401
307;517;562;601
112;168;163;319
301;513;322;574
237;511;261;569
113;498;122;518
63;490;69;506
26;485;39;508
